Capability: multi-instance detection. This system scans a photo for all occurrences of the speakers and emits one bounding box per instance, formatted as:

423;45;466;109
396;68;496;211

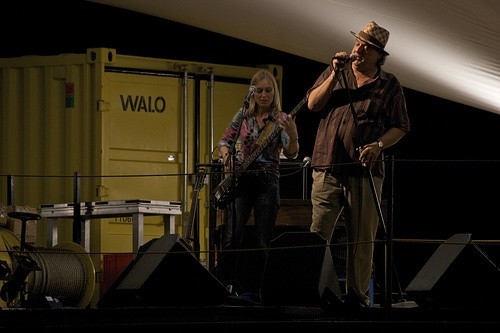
404;233;500;322
94;233;235;308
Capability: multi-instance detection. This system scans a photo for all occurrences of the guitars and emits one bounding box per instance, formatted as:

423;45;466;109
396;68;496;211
210;98;308;212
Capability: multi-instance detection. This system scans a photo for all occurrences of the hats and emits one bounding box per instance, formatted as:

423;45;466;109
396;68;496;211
350;20;390;55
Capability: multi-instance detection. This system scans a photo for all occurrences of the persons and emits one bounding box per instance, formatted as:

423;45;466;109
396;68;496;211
210;68;300;308
303;20;412;310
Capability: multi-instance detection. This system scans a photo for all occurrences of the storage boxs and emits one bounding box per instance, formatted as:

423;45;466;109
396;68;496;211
2;206;38;245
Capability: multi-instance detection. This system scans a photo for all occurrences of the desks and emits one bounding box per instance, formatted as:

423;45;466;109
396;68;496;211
40;199;182;256
213;199;388;307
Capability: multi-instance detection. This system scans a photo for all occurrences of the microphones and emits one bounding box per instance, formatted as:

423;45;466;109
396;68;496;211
332;54;356;62
300;157;312;167
243;86;257;103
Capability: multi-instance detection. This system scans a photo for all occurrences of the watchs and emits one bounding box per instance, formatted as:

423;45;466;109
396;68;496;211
375;139;386;151
288;137;300;145
